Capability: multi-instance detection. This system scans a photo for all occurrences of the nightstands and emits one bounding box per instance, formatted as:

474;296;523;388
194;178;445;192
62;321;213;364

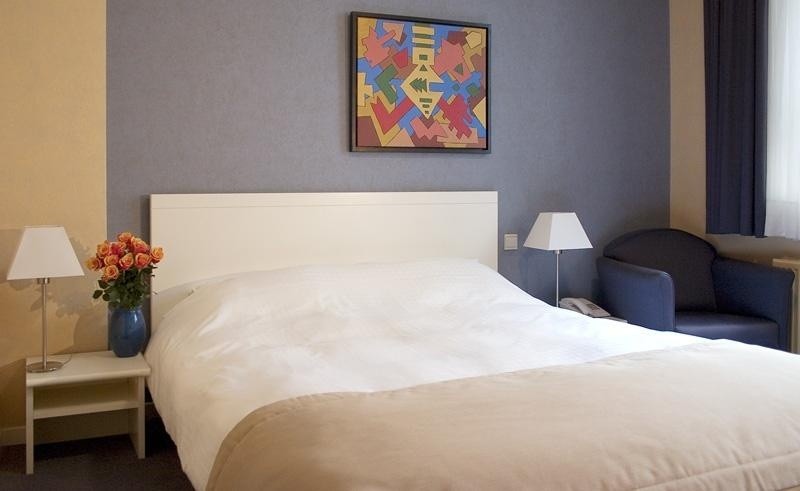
560;306;628;324
24;350;153;476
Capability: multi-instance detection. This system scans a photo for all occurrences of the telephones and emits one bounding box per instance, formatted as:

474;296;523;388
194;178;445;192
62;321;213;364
559;297;611;318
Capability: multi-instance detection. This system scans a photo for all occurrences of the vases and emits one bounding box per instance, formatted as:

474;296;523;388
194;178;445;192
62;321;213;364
110;303;147;357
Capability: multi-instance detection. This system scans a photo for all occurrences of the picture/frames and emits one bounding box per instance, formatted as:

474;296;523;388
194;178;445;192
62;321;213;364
349;10;491;154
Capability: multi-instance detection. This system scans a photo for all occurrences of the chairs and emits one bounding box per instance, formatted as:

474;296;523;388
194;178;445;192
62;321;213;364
595;228;796;354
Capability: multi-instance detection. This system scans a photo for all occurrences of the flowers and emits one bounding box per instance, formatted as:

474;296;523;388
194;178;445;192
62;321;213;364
85;230;165;311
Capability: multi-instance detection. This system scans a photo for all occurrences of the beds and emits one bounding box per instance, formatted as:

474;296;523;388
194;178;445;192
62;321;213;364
148;192;799;491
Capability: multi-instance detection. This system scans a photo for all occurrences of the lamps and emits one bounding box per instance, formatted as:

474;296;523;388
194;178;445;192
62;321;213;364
522;211;594;308
6;226;86;373
